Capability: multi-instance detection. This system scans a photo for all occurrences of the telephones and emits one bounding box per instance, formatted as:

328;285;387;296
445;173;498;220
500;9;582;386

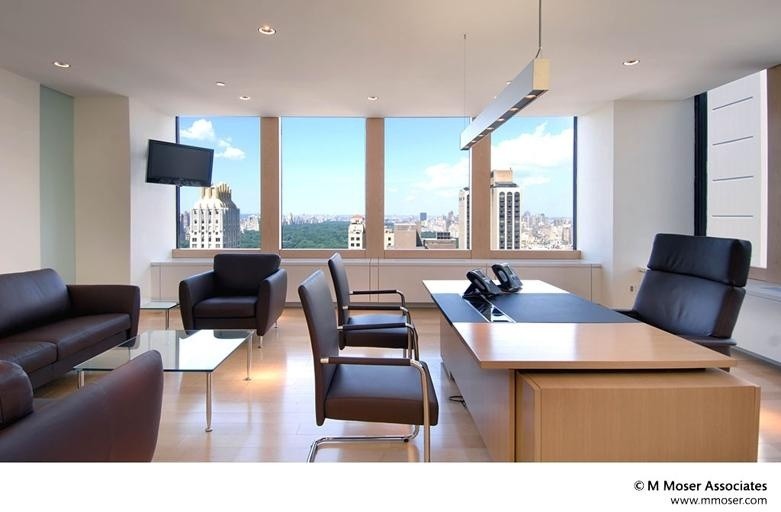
491;263;523;295
462;269;503;300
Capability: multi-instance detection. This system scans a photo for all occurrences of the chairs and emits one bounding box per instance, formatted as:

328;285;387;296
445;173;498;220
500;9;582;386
326;252;420;360
609;230;754;368
297;268;440;462
0;349;166;461
179;253;289;349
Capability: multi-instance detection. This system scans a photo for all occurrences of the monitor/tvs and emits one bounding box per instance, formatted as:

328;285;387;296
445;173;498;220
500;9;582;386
144;138;214;188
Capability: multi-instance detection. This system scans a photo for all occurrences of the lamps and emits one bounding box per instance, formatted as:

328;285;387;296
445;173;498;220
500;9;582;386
456;2;549;156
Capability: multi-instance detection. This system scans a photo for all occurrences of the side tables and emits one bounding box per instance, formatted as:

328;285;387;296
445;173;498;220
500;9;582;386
139;297;179;330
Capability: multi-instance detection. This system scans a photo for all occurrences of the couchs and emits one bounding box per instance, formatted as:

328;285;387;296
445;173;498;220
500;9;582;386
0;268;141;366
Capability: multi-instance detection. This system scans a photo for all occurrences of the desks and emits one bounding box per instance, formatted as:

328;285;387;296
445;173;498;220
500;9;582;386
422;278;762;463
